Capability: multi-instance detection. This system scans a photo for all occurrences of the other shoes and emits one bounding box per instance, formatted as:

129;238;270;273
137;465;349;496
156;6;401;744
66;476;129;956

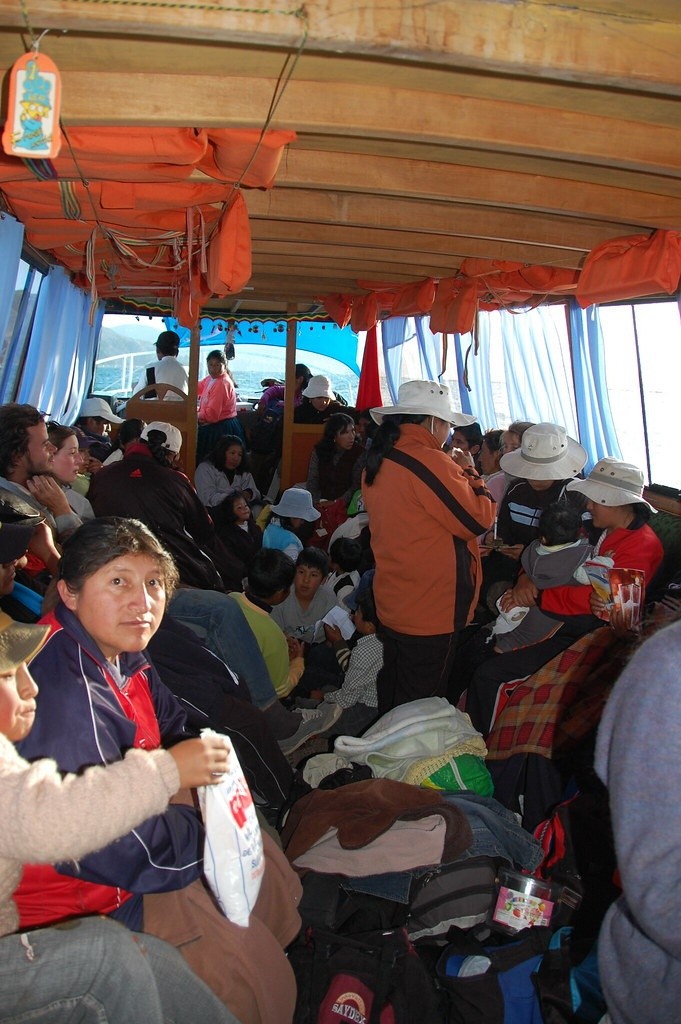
309;687;323;701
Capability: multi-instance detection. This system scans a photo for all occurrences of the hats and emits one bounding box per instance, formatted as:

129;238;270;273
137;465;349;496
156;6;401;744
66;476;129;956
140;421;183;453
500;421;588;480
0;522;34;564
154;331;180;349
0;607;51;673
270;487;321;521
302;374;336;400
369;380;477;428
565;457;659;513
78;397;123;424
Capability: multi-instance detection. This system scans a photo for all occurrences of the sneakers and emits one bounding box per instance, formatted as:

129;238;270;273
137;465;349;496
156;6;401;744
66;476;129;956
275;700;342;756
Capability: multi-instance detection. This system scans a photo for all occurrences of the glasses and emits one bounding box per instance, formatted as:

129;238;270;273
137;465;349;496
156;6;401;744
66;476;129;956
2;549;28;569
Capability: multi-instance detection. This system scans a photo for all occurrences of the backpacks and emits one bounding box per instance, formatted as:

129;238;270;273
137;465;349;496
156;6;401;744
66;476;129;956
407;859;498;931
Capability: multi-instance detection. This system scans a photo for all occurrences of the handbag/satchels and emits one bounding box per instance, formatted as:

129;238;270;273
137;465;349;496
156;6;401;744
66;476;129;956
195;727;265;925
432;922;588;1023
295;927;433;1024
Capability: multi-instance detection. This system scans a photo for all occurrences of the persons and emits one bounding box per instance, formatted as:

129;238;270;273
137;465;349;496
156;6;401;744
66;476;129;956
0;331;681;1024
